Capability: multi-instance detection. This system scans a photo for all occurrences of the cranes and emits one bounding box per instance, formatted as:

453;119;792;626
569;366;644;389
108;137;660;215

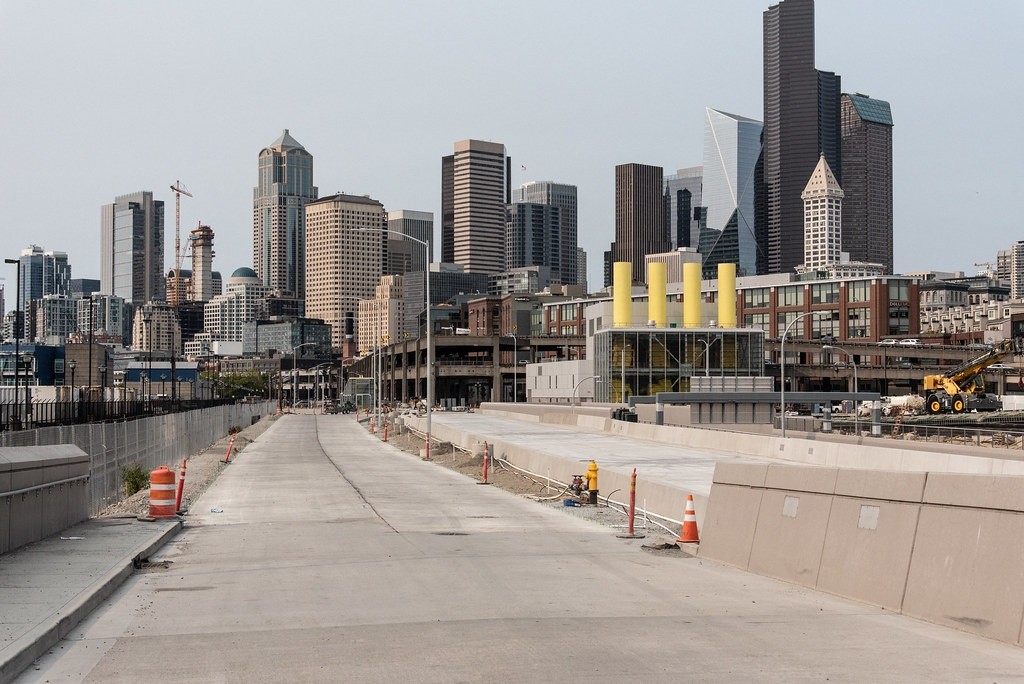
171;179;195;307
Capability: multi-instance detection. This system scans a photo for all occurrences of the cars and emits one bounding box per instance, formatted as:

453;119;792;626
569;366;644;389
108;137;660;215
323;403;351;415
519;359;531;366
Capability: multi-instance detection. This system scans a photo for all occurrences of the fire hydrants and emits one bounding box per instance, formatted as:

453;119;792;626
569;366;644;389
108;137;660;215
586;460;603;505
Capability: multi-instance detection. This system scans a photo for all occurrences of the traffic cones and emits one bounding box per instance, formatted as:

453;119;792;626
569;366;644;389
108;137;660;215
676;494;699;543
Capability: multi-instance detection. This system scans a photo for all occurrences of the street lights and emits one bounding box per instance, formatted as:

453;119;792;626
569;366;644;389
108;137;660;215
210;381;216;399
139;369;147;417
200;381;205;402
822;345;858;436
595;380;618;402
290;227;432;441
22;352;32;430
505;333;518;405
697;339;709;377
122;368;129;421
4;258;20;418
204;346;217;400
142;317;155;414
98;364;108;420
558;346;581;404
189;378;194;400
68;359;77;423
82;295;93;418
166;331;175;404
160;373;167;401
781;311;831;440
571;375;602;405
177;376;183;401
621;344;630;403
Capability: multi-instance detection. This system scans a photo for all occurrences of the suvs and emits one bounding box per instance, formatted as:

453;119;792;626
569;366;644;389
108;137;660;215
876;339;898;346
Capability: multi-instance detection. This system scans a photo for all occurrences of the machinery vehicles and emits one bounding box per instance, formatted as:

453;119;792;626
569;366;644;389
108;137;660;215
918;337;1024;416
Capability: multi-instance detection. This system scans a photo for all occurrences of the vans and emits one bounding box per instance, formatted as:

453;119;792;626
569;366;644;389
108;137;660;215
899;339;921;345
